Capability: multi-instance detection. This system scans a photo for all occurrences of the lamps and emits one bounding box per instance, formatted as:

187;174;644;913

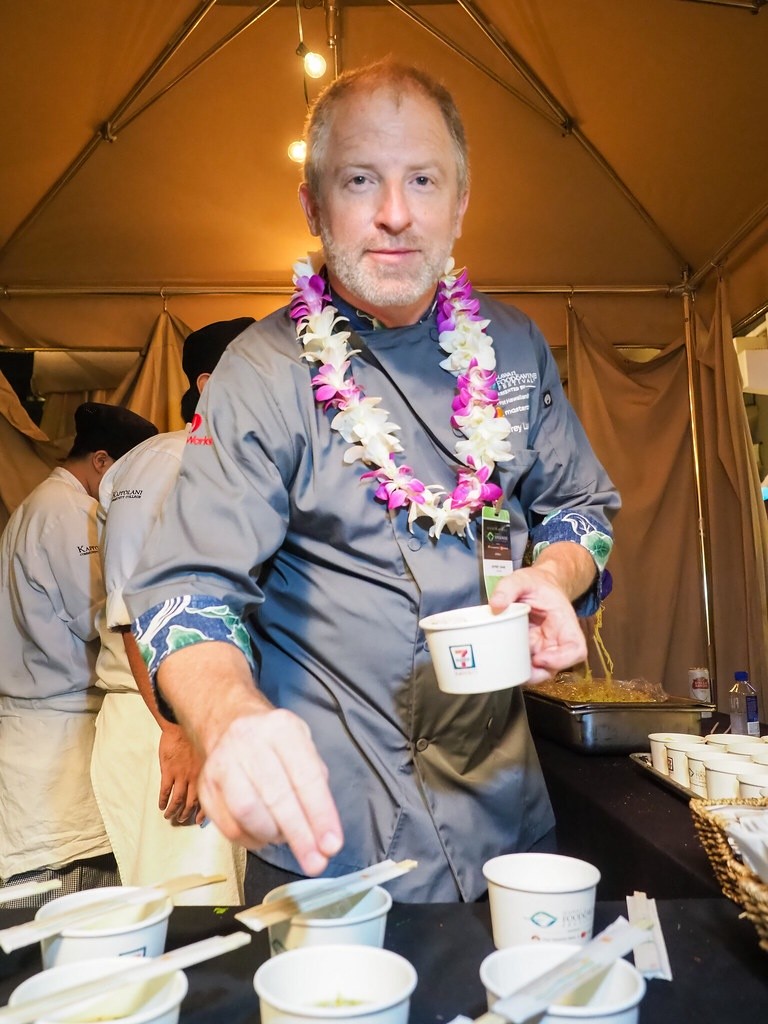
296;0;327;79
287;141;307;162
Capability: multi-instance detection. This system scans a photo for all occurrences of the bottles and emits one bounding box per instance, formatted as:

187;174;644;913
727;671;760;738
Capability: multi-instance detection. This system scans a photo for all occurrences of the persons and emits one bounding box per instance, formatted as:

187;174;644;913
1;404;161;861
124;62;622;907
88;317;257;907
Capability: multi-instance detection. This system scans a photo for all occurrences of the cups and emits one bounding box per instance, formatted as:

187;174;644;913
646;731;768;802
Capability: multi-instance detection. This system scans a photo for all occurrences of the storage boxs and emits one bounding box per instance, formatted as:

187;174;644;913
524;686;718;748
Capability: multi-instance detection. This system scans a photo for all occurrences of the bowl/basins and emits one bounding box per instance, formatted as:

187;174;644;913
252;945;419;1024
263;880;392;962
482;851;602;947
419;603;533;697
479;940;648;1023
35;886;173;971
8;959;190;1024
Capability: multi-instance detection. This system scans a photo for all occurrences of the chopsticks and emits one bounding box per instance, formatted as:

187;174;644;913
233;858;418;932
0;931;252;1024
0;874;228;954
632;891;662;981
472;914;656;1022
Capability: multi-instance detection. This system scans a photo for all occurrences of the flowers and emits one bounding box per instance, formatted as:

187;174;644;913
288;247;519;542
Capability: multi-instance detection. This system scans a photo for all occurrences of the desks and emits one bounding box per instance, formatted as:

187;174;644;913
0;896;767;1024
536;709;767;899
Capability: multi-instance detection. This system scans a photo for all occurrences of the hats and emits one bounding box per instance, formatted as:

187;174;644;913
182;314;257;376
76;400;161;450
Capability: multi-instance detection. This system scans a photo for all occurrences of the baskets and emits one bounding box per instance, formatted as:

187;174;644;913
686;793;767;952
